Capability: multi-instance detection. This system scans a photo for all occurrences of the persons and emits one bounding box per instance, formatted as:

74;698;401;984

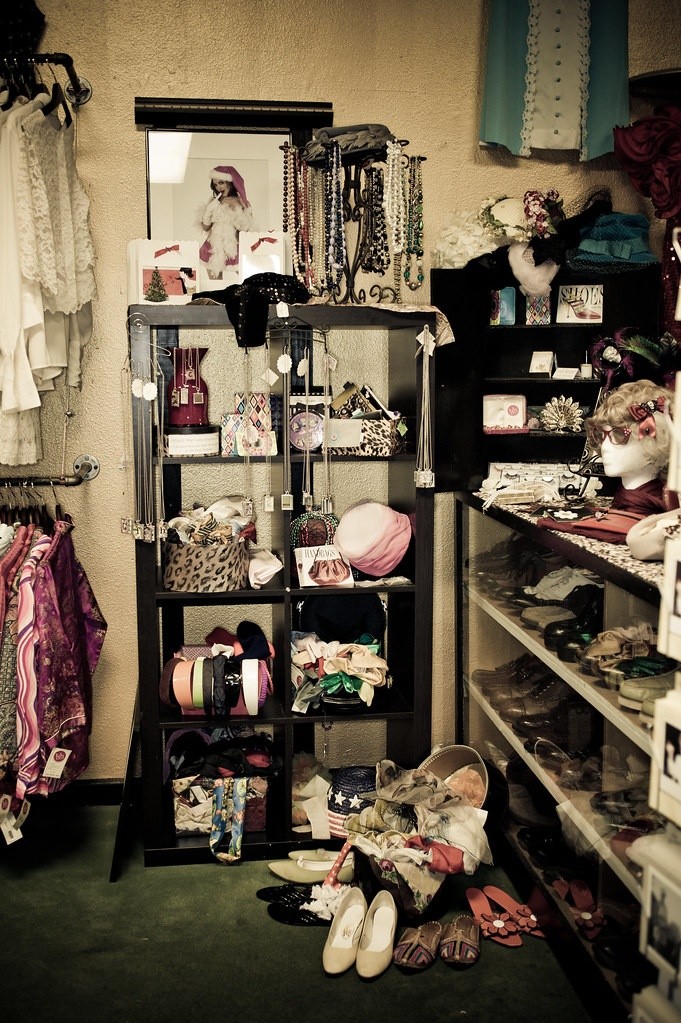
587;380;674;491
200;166;253;280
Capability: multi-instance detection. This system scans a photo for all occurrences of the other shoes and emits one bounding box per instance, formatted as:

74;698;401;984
464;535;681;728
268;858;354;883
256;882;318;905
472;650;668;832
266;902;334;927
289;849;354;864
503;758;660;1002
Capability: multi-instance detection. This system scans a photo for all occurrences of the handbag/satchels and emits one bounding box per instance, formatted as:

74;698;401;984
334;500;412;577
626;506;681;560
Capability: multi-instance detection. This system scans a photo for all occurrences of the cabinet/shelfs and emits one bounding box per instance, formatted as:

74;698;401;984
445;489;681;1021
429;248;669;493
124;302;437;871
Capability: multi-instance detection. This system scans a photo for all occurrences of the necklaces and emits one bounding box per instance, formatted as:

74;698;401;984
414;325;435;487
171;347;204;407
242;346;333;516
283;141;424;302
121;360;167;542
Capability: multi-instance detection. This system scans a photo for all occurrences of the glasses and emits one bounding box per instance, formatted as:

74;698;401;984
590;427;633;445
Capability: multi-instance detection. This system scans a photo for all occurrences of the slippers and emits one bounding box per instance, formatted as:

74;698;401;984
484;886;551;937
440;913;481;964
466;887;522;946
393;921;442;969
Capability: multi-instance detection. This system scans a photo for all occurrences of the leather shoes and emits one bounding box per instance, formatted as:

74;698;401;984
322;887;367;974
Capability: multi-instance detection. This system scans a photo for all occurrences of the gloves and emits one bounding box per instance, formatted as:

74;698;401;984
202;193;222;225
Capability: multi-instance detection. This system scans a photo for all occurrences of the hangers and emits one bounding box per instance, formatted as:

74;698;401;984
0;476;70;539
0;52;73;135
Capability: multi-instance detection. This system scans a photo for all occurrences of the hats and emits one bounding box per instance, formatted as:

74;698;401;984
209;166;252;214
571;211;660;264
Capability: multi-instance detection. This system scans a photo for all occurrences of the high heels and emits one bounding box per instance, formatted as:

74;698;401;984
357;890;397;978
